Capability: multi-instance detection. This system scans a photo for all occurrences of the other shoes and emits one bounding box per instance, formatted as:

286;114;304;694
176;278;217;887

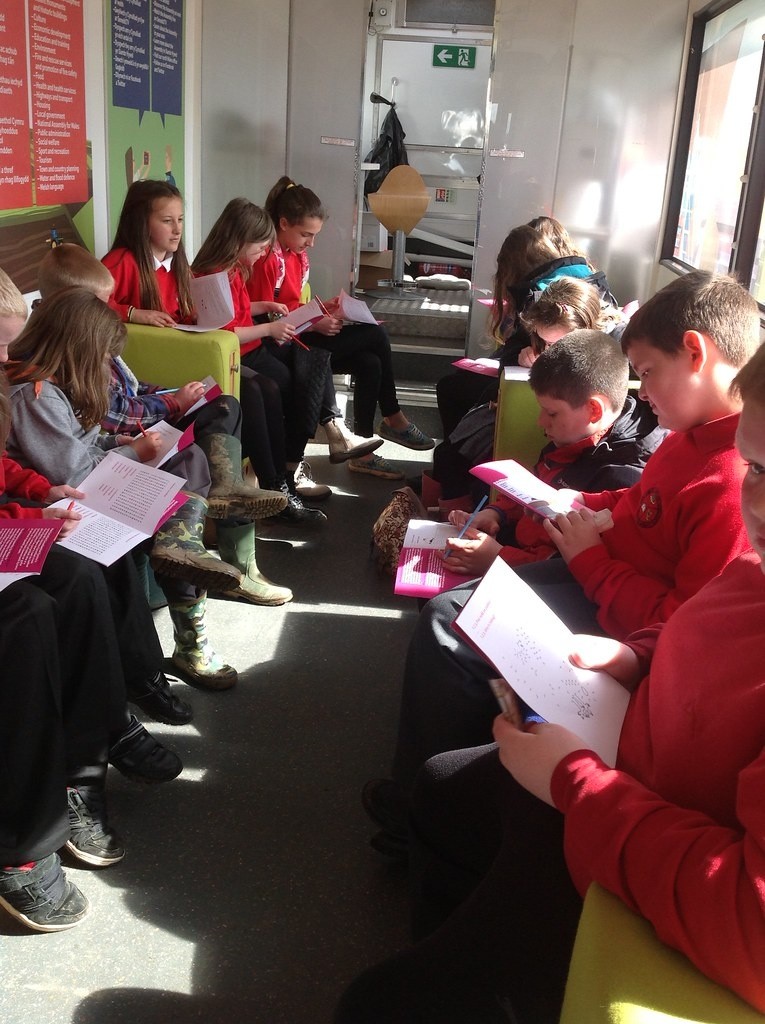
363;777;411;830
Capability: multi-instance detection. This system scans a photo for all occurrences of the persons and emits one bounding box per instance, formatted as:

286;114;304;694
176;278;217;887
340;341;764;1024
364;216;764;821
0;242;297;933
101;176;434;528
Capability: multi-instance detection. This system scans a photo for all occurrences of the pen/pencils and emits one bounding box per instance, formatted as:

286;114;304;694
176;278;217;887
532;335;536;356
156;384;206;395
136;420;146;436
449;598;461;610
290;334;310;351
67;500;75;511
443;495;488;560
316;295;331;318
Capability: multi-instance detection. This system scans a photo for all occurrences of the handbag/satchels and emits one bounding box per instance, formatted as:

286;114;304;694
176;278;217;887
362;486;431;577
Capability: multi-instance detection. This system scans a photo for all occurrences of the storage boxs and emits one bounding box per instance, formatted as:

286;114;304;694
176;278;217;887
360;211;389;253
357;249;392;289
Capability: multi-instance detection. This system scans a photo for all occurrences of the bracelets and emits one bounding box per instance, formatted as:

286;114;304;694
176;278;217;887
128;306;135;322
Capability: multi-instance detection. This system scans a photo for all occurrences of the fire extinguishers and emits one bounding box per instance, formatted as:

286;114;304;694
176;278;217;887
418;262;471;281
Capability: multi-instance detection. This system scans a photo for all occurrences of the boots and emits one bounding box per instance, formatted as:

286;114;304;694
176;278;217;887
171;591;238;688
324;417;384;464
421;469;442;520
439;494;474;522
260;477;328;527
151;490;240;590
216;520;293;605
197;433;287;519
285;461;333;500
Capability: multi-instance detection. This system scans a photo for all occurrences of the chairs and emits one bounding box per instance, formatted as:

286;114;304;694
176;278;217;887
367;166;433;288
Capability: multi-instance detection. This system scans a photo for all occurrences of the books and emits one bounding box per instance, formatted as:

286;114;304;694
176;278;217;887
395;519;479;598
335;289;393;325
185;377;222;416
471;460;598;526
0;517;65;592
47;452;190;567
134;418;196;468
274;299;324;346
171;272;235;332
453;556;630;770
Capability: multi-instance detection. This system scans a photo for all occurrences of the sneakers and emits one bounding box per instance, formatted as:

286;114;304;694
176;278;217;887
378;420;435;451
348;455;405;478
63;787;126;865
0;852;87;932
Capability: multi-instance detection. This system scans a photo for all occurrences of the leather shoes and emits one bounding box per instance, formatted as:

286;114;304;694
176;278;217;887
108;714;183;781
127;670;194;725
